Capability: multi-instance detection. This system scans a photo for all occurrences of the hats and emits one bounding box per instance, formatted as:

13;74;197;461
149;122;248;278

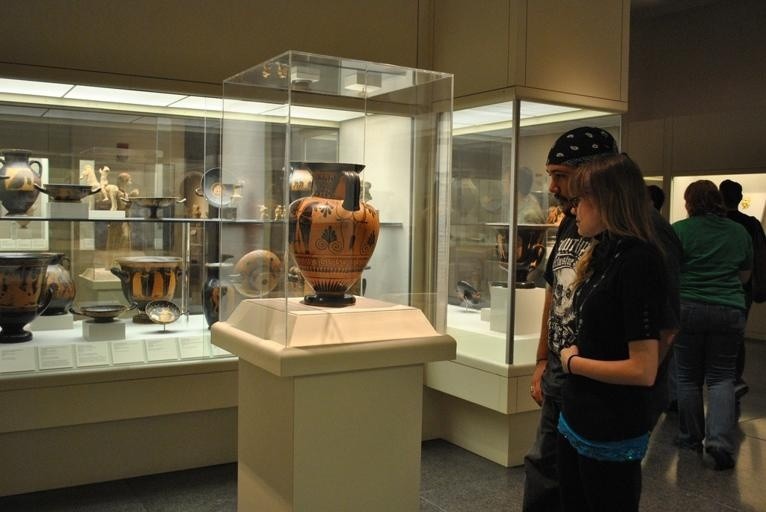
545;125;617;164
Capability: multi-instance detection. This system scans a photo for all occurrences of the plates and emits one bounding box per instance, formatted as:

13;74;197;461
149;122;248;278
232;249;282;297
130;197;179;208
200;167;240;209
43;183;93;202
145;299;181;324
80;304;127;322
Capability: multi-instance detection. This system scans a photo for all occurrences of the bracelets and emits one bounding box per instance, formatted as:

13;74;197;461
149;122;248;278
566;352;579;374
534;357;549;366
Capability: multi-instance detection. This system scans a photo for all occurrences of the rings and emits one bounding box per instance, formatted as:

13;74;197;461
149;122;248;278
530;385;535;392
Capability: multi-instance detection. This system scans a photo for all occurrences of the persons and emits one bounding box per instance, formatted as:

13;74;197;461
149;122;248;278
107;173;140;250
717;179;766;386
645;185;679;418
520;124;621;510
499;164;545;226
669;179;755;471
557;150;685;510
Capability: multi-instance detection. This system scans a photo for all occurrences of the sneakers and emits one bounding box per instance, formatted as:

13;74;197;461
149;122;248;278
704;438;736;470
732;376;750;399
673;435;704;450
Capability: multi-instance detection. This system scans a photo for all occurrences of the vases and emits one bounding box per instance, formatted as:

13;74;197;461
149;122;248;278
42;253;76;316
0;253;57;343
289;162;380;307
486;222;560;288
201;263;237;330
110;257;185;325
0;149;42;217
